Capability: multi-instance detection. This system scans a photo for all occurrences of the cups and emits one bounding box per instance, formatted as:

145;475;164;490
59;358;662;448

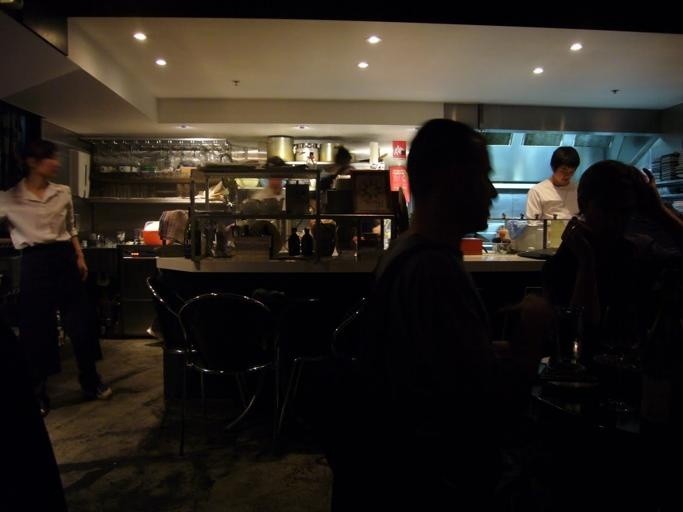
242;199;257;214
100;165;130;172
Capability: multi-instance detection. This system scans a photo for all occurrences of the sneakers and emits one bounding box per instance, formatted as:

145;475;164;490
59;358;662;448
79;379;113;401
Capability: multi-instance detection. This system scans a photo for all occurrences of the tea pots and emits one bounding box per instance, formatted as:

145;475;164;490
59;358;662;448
256;197;285;215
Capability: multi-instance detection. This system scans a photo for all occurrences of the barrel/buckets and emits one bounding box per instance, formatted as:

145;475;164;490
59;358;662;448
285;181;310;216
460;237;484;256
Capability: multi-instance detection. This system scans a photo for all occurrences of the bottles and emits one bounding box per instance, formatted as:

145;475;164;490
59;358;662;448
184;221;224;259
288;219;381;257
641;298;683;426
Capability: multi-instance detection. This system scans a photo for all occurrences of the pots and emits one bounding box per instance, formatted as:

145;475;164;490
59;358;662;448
266;136;343;162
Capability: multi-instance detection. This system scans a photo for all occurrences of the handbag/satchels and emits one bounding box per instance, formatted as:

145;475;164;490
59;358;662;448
331;241;448;370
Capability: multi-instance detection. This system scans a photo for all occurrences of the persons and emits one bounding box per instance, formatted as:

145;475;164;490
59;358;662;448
226;148;336;254
323;113;562;511
308;142;386;248
519;140;588;221
0;137;114;418
539;158;682;438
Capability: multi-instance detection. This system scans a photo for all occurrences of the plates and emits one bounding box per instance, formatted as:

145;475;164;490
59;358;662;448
652;153;683;182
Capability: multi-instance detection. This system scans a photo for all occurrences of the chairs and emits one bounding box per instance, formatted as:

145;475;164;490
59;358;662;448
174;294;275;451
332;298;369;412
274;293;338;440
146;275;185;433
